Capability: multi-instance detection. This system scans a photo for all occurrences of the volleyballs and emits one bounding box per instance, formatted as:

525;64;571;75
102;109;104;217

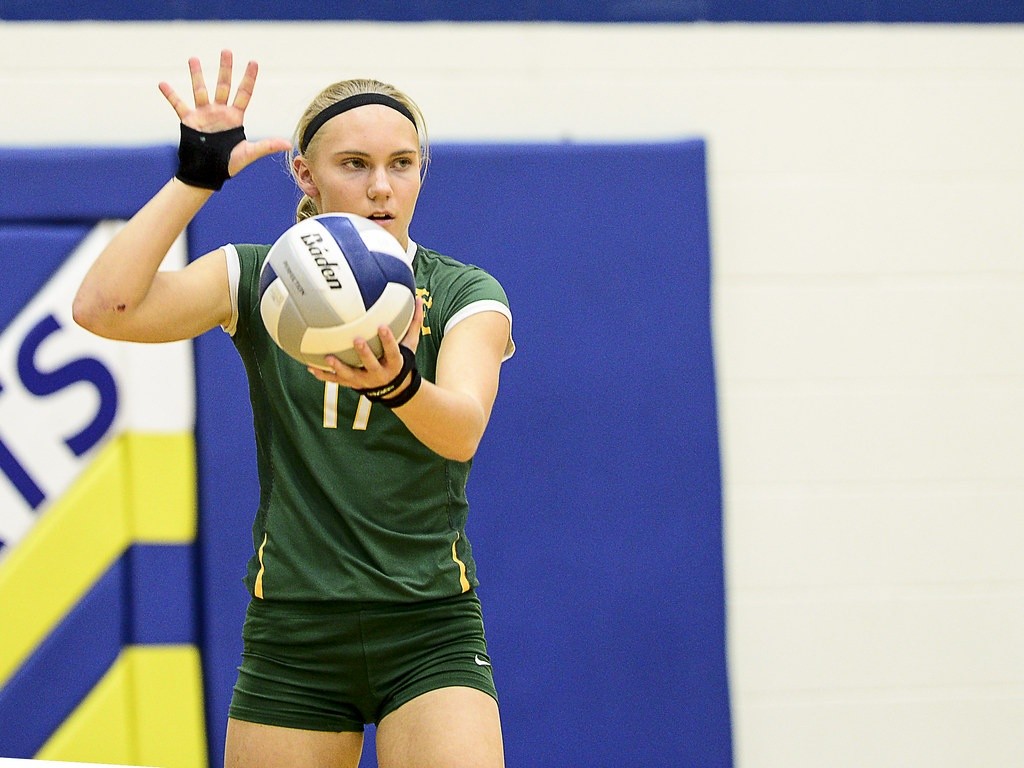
259;211;418;374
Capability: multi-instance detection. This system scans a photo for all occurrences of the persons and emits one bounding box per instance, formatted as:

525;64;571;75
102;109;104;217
67;48;515;768
